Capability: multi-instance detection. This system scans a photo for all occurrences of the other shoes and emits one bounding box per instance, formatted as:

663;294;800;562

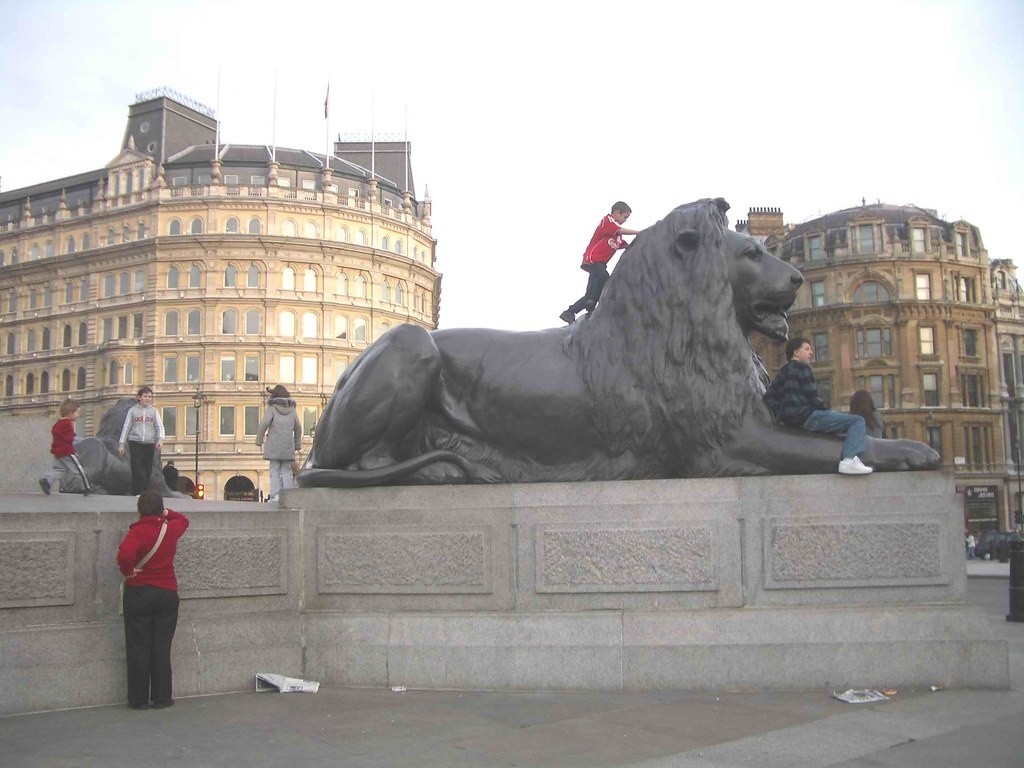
132;488;145;496
126;699;176;710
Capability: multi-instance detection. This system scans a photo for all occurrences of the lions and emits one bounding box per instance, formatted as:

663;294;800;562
58;398;192;498
295;194;941;489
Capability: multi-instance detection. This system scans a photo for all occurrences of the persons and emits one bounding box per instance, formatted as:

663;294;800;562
117;385;166;498
39;399;103;497
965;528;982;560
762;337;875;475
255;385;303;503
117;490;189;709
850;391;886;440
162;460;179;490
558;201;661;324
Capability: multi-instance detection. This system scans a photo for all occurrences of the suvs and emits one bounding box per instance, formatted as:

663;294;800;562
974;531;1024;560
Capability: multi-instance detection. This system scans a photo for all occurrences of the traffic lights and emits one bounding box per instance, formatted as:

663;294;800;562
197;484;204;499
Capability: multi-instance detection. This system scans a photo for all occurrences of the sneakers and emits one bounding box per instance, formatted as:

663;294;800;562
83;485;101;496
837;455;873;474
39;478;50;495
585;301;594;312
559;310;575;323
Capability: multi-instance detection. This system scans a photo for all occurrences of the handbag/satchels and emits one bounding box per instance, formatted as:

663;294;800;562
118;581;124;617
291;449;301;472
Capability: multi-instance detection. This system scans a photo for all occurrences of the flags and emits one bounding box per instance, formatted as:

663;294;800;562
324;83;329;119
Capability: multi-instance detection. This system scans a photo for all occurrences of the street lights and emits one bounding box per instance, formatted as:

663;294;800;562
309;426;315;444
192;385;207;499
926;411;937;448
1013;438;1023;532
